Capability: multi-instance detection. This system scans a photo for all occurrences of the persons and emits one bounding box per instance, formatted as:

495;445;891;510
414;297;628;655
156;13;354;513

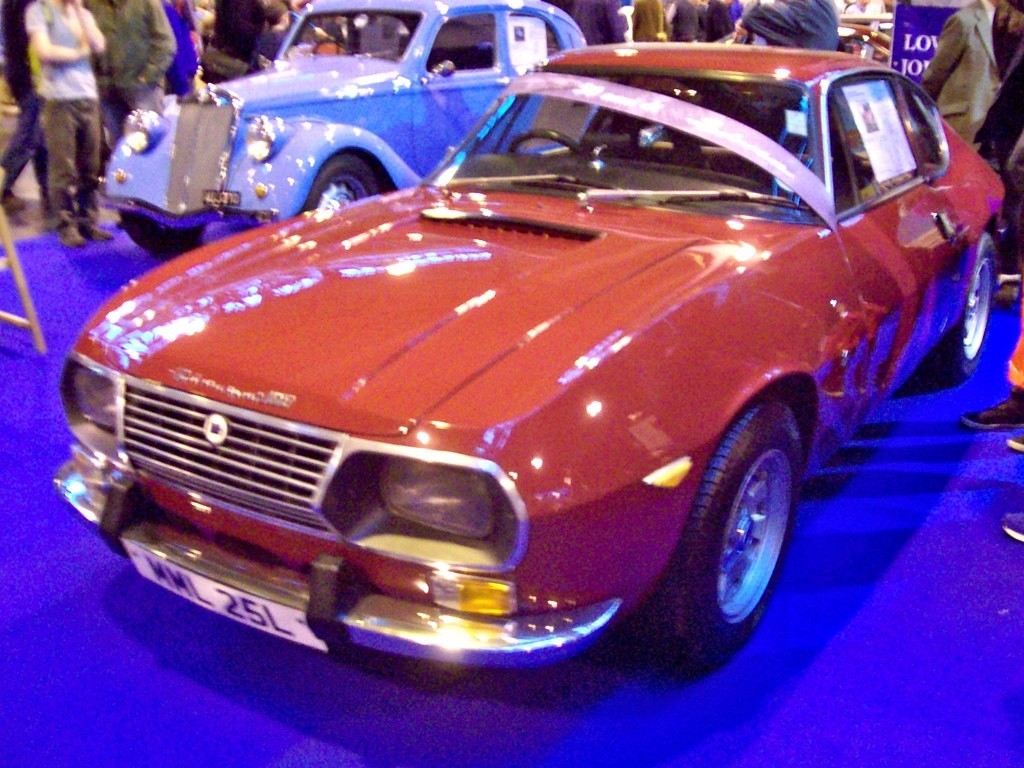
0;0;367;249
547;0;1024;542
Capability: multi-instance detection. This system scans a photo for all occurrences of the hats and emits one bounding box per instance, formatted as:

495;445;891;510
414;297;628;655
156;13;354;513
264;0;287;23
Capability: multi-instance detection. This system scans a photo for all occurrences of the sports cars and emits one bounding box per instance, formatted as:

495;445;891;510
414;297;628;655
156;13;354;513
96;1;646;253
44;39;1006;678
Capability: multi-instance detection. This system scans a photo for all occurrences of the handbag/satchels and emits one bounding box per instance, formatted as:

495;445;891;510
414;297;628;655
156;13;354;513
201;45;250;81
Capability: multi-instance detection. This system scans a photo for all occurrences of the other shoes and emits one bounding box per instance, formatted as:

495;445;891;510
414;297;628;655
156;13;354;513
58;224;86;247
1006;432;1024;452
1001;510;1024;542
994;282;1019;308
961;385;1024;428
0;189;27;212
78;221;113;241
